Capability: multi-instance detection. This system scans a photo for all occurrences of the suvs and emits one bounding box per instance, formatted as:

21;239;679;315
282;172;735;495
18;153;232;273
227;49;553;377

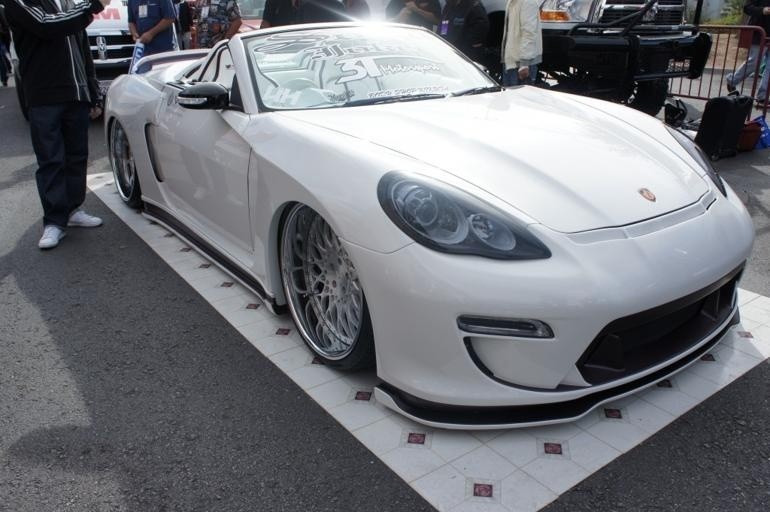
364;0;714;118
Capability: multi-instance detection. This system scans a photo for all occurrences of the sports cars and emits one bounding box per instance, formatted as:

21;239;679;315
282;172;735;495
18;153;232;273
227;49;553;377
104;19;756;433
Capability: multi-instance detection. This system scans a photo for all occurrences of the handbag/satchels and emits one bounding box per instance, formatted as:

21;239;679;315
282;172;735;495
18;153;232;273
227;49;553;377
738;120;761;151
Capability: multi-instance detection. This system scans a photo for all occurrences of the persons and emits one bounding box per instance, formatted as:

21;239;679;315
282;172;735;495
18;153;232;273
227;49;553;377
500;0;543;86
0;0;112;249
128;0;243;56
260;0;490;50
726;0;770;106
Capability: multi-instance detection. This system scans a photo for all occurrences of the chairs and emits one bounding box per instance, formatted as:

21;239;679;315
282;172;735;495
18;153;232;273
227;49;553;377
189;35;382;111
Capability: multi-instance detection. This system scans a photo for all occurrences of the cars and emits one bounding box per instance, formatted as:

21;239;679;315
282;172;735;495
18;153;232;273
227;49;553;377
86;0;182;100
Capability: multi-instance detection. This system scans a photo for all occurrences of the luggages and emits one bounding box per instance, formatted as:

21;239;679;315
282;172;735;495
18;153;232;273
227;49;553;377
693;90;753;162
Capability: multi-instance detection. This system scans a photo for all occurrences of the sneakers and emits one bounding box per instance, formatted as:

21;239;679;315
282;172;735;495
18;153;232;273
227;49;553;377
39;225;67;248
726;74;736;91
66;210;103;227
755;99;770;108
